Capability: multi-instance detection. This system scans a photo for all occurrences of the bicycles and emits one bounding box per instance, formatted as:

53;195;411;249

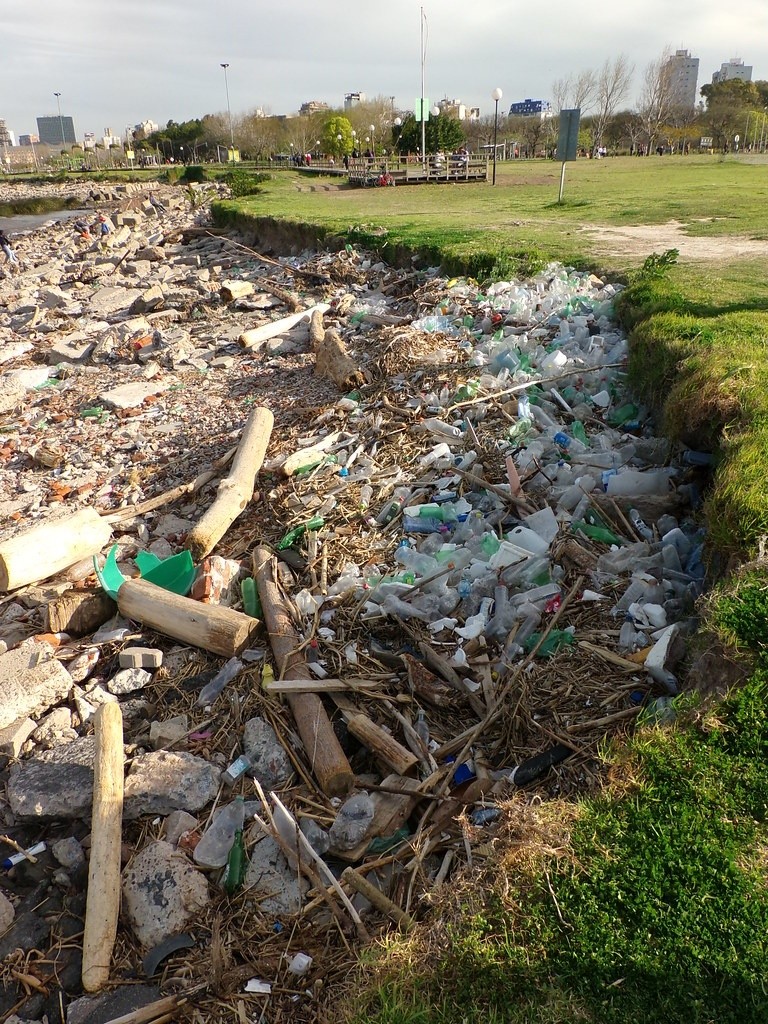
359;164;386;188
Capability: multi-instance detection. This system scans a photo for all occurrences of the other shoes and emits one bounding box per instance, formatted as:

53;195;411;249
436;172;440;176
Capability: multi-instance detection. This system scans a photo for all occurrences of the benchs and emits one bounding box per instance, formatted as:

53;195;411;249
444;164;484;175
368;170;408;177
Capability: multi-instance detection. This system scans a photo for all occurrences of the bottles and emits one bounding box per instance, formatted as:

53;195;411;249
91;243;705;1024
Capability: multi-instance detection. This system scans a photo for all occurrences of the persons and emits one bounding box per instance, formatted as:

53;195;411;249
452;147;470;175
433;151;444;175
73;210;110;240
0;230;18;262
685;142;690;155
637;143;647;155
365;149;373;171
515;148;557;159
660;143;664;156
343;154;349;169
596;146;606;158
724;140;729;154
150;192;167;212
748;142;752;153
289;154;310;166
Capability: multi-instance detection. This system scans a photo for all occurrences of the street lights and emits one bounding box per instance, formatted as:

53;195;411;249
431;107;440;153
390;96;395;108
394;117;402;169
369;125;375;151
54;92;66;150
351;130;370;150
220;63;234;145
290;134;342;159
492;88;502;185
